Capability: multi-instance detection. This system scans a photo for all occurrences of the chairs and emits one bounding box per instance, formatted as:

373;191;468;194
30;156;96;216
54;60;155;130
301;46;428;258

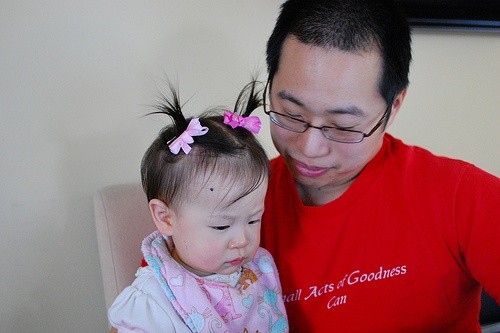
93;181;162;327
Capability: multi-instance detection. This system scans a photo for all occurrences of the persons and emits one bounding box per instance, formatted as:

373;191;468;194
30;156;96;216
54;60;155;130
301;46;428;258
106;71;291;332
140;0;500;332
